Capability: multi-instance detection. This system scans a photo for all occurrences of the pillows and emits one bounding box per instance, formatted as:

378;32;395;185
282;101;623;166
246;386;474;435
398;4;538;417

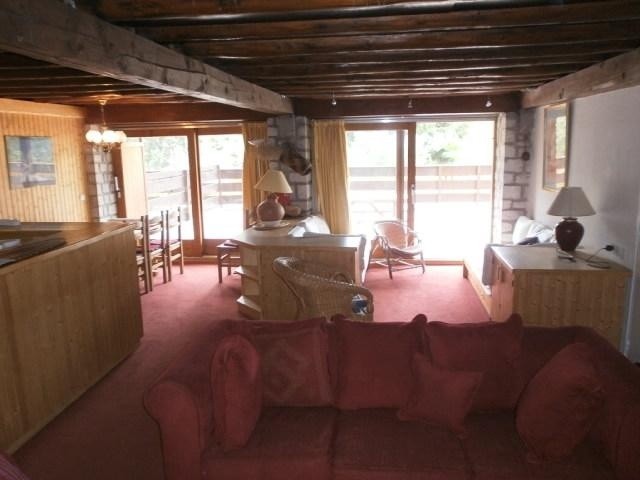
330;308;426;413
510;339;615;462
241;316;336;415
206;333;264;453
397;349;487;439
419;309;526;418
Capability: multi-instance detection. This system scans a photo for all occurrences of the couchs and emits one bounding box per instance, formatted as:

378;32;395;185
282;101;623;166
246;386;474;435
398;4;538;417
463;216;585;318
142;319;640;480
288;213;371;284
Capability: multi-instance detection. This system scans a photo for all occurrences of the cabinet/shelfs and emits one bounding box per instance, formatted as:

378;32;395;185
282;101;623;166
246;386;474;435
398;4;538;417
490;244;634;352
231;216;362;322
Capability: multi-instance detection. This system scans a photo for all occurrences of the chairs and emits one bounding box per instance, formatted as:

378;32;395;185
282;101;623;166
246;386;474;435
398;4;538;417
369;219;425;280
165;206;184;282
215;206;257;283
145;211;170;292
272;256;374;322
122;216;148;298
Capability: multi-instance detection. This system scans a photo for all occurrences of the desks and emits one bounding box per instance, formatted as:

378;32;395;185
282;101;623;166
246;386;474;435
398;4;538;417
0;222;143;455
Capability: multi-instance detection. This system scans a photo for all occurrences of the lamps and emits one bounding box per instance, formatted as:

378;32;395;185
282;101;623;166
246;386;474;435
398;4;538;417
548;185;596;259
485;99;493;108
85;125;127;155
252;168;295;230
406;101;414;108
332;98;337;106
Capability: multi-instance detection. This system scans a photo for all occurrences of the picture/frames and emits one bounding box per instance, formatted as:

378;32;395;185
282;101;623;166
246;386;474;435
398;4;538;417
542;99;571;194
4;136;57;191
